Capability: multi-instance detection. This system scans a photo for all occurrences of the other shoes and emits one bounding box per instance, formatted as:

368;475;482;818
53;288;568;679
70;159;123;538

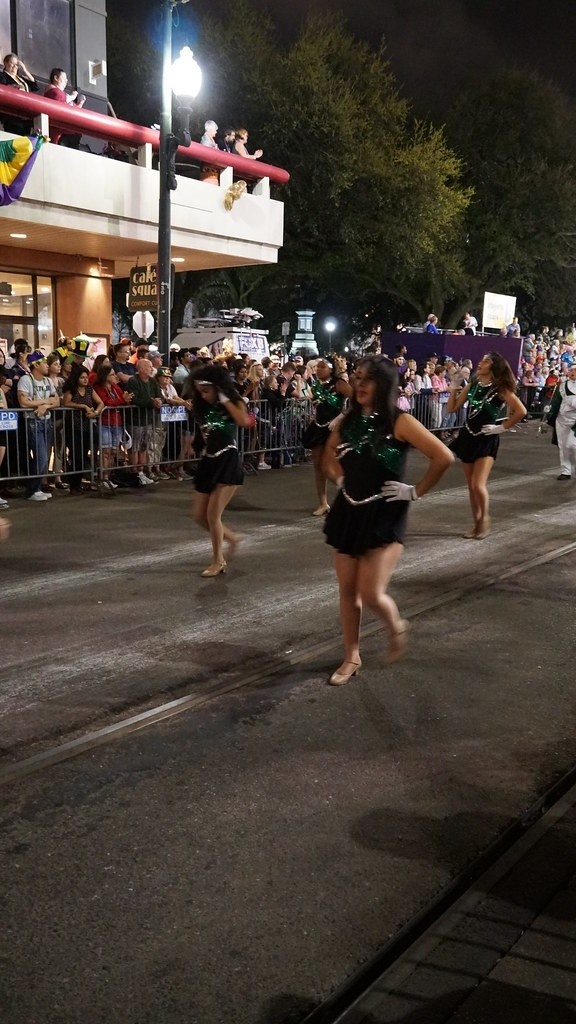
42;457;272;496
557;474;571;480
0;498;7;504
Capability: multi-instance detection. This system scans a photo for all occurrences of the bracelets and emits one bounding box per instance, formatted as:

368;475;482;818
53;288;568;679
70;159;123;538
410;487;416;501
342;411;345;416
94;410;98;415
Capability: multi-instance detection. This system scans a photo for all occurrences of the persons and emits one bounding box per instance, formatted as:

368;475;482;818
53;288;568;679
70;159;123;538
450;349;530;540
44;68;87;151
217;349;314;472
0;53;40;137
311;355;355;517
424;310;478;337
0;331;199;512
216;124;236;155
200;120;220;186
321;354;454;687
230;127;264;195
390;343;474;441
101;140;130;163
186;365;251;577
543;364;576;481
502;313;576;419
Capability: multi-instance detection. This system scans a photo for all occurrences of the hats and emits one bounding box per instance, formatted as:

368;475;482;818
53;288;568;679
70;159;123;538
269;355;281;363
295;356;303;362
155;367;172;377
27;350;47;367
52;347;74;365
71;335;90;357
148;351;165;360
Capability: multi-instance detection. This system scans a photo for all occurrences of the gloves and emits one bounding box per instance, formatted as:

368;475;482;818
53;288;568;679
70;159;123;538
450;368;465;389
481;424;509;435
381;480;422;502
218;391;230;405
336;475;345;490
328;412;346;432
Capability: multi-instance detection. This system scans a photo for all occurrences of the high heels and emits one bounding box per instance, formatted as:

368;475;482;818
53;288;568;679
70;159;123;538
312;504;330;515
385;619;412;663
330;656;362;685
463;516;492;539
201;560;227;577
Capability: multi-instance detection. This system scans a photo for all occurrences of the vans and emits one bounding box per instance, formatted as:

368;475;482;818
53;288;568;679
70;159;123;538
168;305;270;366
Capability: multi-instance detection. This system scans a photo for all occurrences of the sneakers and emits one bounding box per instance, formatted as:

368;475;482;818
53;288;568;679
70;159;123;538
27;491;52;501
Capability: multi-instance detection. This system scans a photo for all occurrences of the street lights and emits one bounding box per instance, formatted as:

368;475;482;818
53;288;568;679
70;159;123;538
323;317;337;352
154;0;206;369
